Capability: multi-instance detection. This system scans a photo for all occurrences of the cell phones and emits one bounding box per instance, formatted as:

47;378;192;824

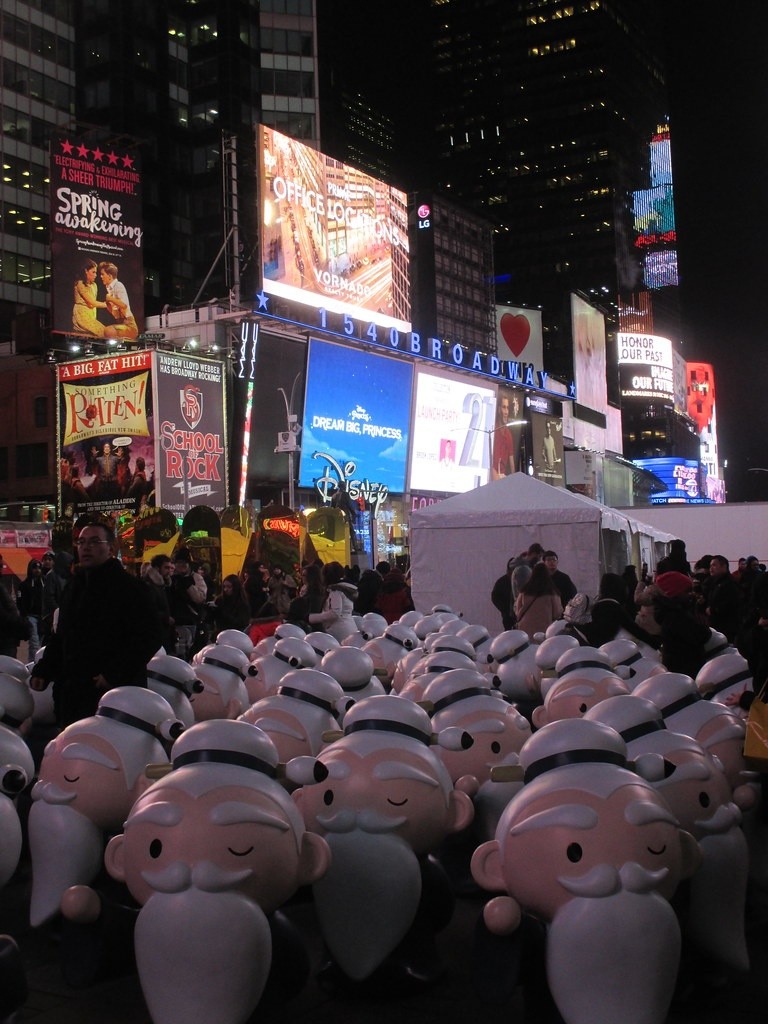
643;563;648;573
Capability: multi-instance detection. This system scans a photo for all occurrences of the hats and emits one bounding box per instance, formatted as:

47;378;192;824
172;547;190;563
656;571;695;598
42;551;55;562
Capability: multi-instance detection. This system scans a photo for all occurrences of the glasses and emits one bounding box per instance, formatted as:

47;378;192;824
72;538;108;546
33;565;41;570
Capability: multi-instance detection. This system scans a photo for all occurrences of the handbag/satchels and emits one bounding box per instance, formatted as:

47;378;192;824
743;696;768;761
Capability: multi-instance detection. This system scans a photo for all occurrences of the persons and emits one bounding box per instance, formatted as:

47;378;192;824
541;421;558;486
0;607;768;1024
0;520;768;760
60;258;155;516
490;395;515;481
